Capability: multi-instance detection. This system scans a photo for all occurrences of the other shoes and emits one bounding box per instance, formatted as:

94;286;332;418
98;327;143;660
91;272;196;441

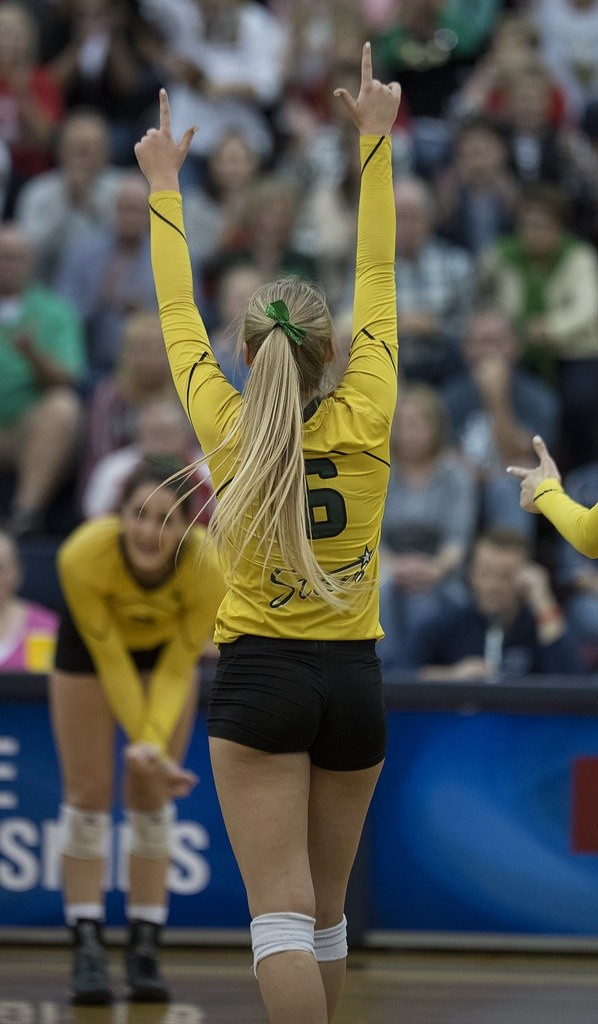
68;942;110;1006
121;940;173;1001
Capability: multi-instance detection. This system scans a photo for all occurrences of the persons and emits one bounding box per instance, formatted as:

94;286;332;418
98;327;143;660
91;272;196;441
1;0;598;1010
134;39;405;1024
